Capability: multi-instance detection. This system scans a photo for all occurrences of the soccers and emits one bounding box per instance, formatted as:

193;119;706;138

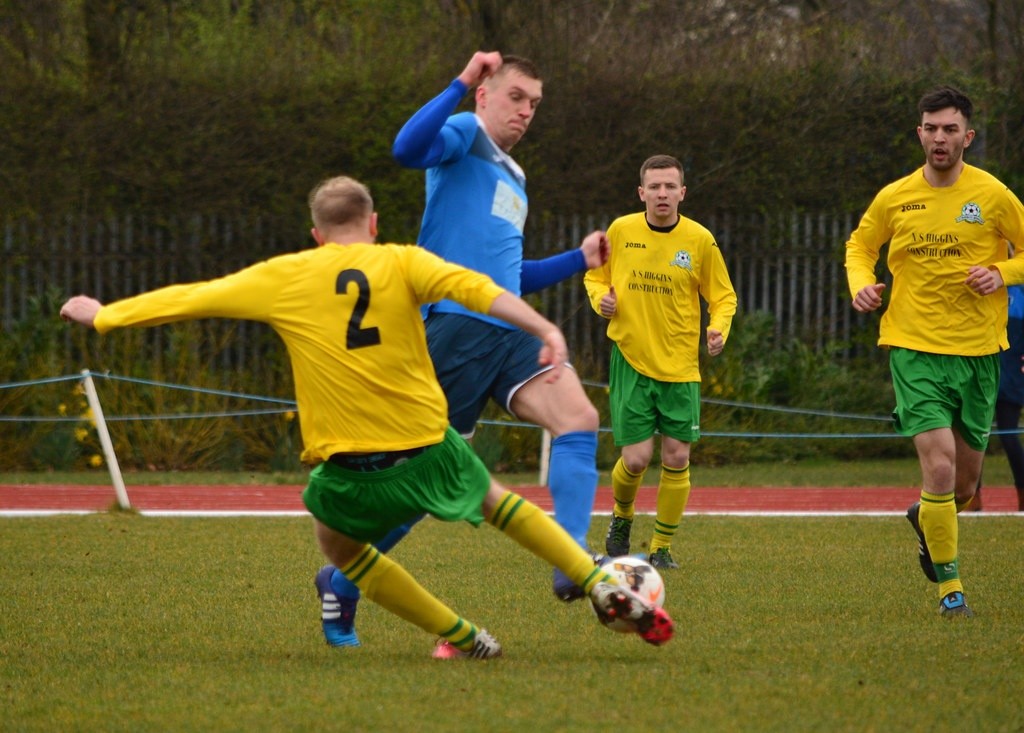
592;554;666;633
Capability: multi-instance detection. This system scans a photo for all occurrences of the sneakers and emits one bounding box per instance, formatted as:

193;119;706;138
605;512;633;557
432;627;501;659
554;553;648;602
649;547;677;570
314;565;361;647
590;581;674;646
939;592;972;620
907;500;942;584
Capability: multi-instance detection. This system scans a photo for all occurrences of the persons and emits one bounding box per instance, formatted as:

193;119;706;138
60;176;677;662
964;284;1024;513
583;154;737;569
313;50;648;645
846;84;1024;616
